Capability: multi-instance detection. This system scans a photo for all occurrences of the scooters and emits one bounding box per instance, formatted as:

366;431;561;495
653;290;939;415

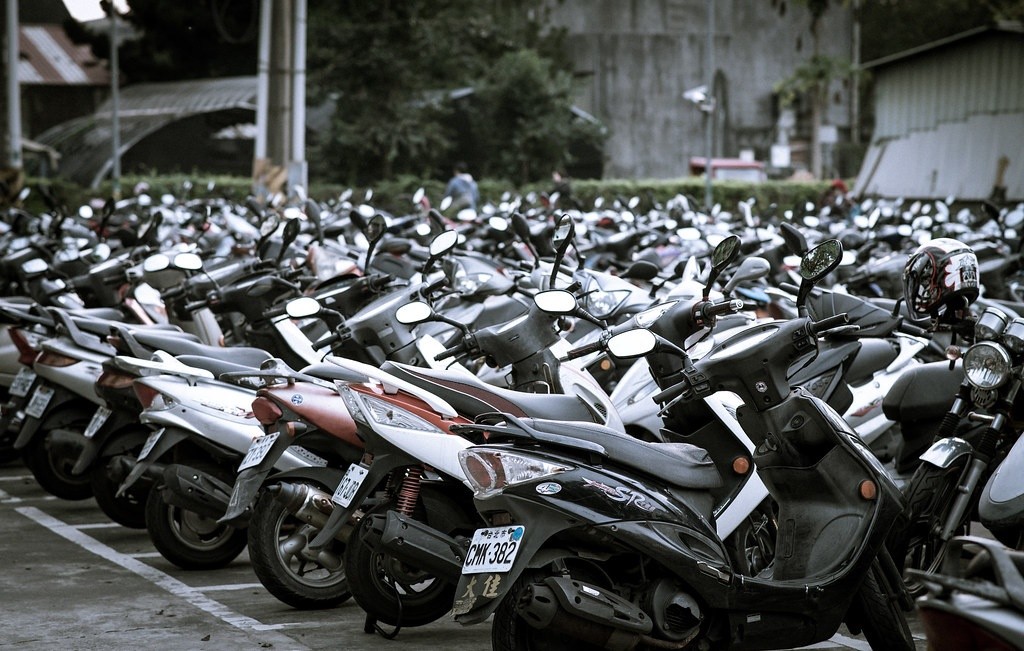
0;181;1024;651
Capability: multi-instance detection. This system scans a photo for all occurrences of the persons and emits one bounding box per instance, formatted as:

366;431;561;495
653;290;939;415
441;161;481;220
544;169;578;211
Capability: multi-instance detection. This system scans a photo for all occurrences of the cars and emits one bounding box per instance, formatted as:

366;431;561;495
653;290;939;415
689;159;768;182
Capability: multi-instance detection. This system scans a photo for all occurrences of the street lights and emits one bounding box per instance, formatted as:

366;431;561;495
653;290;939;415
101;0;131;196
